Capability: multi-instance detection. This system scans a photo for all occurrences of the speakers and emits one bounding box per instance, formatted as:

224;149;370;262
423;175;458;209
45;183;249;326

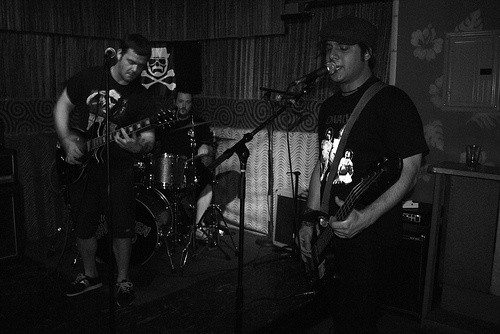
0;153;21;264
272;186;307;250
382;232;428;320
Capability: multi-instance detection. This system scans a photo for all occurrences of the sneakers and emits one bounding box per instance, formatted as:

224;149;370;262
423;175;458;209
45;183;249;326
64;272;105;296
114;277;135;307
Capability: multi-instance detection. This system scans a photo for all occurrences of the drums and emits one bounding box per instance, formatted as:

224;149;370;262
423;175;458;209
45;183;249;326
152;151;188;191
95;183;175;267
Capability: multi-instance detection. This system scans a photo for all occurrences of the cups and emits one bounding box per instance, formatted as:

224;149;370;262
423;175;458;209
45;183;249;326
465;145;481;168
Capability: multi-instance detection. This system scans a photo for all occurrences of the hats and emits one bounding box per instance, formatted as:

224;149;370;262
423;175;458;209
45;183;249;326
318;15;379;49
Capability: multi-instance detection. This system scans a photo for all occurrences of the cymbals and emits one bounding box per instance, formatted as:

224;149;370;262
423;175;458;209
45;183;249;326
196;135;238;144
171;119;217;131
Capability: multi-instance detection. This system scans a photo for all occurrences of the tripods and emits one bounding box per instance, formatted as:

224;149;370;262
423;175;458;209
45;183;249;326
169;128;239;277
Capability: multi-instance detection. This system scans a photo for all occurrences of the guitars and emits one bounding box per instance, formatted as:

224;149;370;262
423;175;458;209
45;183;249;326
273;158;401;319
38;104;180;187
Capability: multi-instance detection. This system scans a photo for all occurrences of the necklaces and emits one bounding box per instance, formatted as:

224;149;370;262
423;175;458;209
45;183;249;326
341;88;360;94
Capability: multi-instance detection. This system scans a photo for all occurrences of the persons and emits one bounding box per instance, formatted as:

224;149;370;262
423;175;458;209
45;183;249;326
157;87;216;239
53;35;157;305
295;15;427;334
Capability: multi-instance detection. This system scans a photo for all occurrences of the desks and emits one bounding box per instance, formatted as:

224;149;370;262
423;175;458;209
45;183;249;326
421;160;500;334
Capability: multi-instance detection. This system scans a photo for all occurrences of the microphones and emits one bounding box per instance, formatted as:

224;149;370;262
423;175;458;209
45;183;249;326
290;61;337;88
104;48;116;60
275;94;296;101
